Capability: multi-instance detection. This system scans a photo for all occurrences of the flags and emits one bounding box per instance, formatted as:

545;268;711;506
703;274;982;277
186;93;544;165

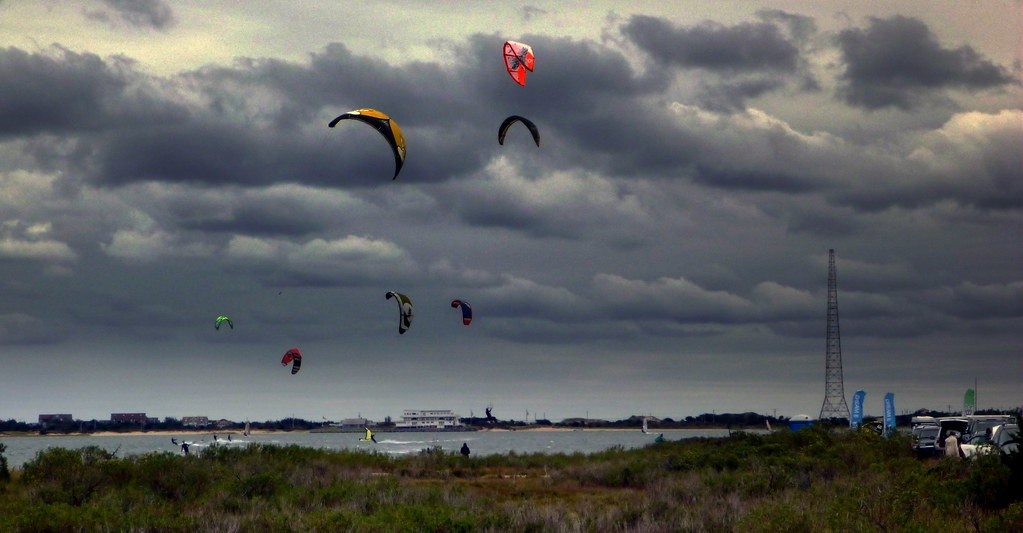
883;393;896;432
850;391;866;428
961;378;975;416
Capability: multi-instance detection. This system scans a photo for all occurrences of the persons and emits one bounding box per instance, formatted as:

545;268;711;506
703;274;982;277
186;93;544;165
486;407;498;423
944;431;959;457
984;428;991;436
171;437;178;445
461;443;470;458
181;440;189;455
